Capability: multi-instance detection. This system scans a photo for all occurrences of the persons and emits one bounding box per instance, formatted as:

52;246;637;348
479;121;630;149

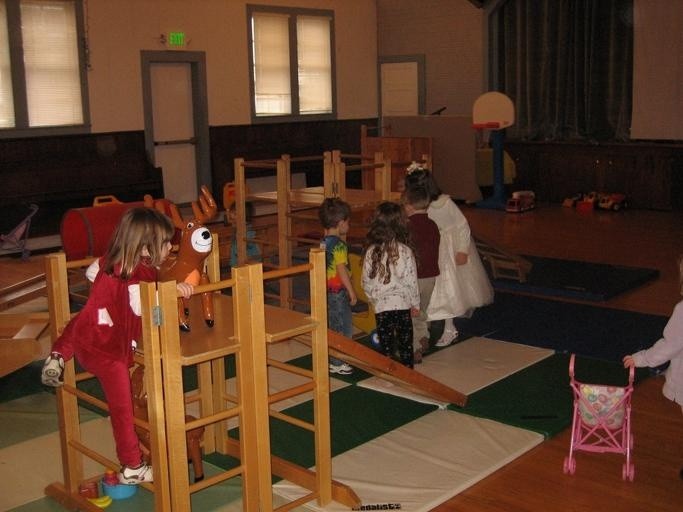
41;208;194;484
360;202;419;370
228;203;261;271
402;169;495;348
316;197;356;375
623;254;683;406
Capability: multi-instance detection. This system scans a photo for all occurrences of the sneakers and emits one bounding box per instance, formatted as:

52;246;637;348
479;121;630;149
119;461;153;486
41;354;65;387
329;362;353;376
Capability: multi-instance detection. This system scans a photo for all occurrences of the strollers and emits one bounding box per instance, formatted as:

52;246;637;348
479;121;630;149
0;202;38;261
558;352;639;485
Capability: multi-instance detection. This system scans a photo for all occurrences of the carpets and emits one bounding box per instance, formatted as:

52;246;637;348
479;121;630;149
486;253;659;303
0;311;648;512
471;291;671;375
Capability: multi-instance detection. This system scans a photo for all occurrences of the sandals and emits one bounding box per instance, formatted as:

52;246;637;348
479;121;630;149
435;328;459;346
